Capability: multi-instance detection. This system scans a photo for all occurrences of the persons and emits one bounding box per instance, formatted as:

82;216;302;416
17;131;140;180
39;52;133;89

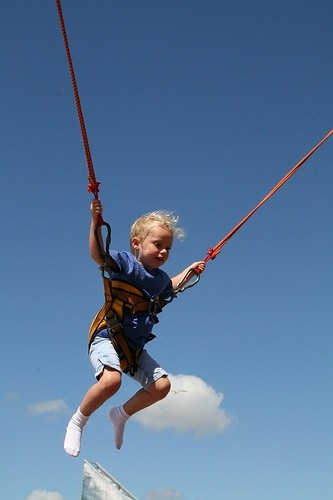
58;200;203;458
